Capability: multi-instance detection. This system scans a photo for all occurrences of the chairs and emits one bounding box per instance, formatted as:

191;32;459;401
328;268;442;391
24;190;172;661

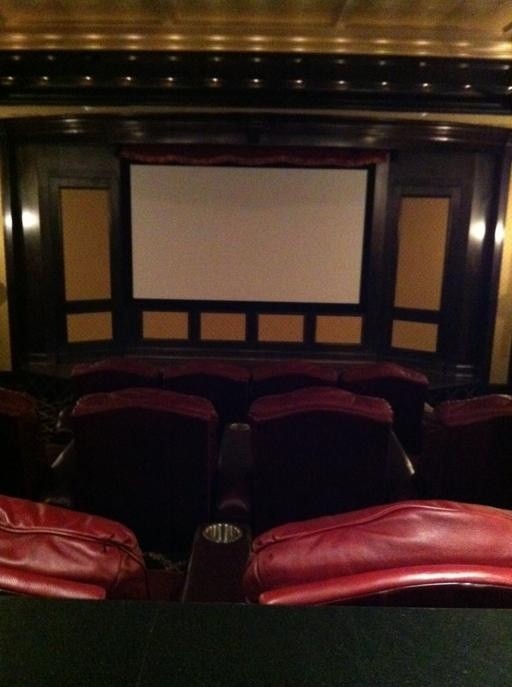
1;494;178;601
72;386;247;532
421;392;510;506
255;359;338;392
340;357;431;475
166;358;253;401
236;499;511;605
1;389;69;500
251;389;417;520
33;356;163;396
